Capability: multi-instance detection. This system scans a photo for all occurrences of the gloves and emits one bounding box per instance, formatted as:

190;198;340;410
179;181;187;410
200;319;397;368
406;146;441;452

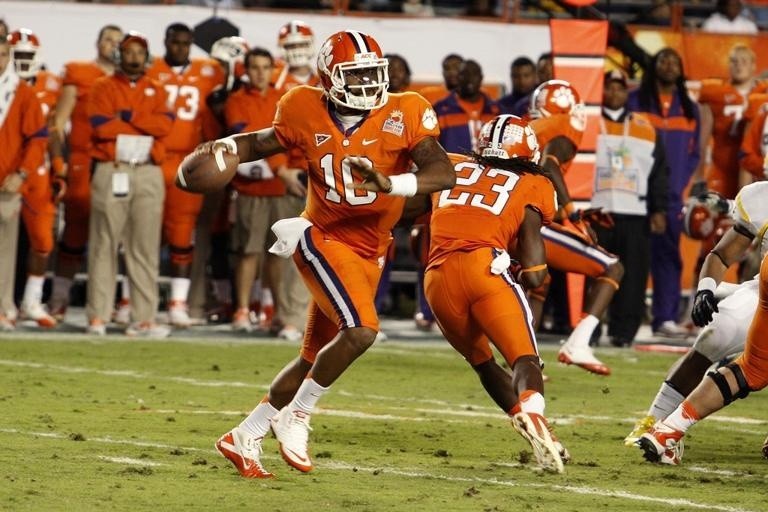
691;290;719;328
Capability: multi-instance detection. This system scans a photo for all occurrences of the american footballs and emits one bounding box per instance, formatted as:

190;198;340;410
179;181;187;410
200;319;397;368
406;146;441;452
173;150;239;194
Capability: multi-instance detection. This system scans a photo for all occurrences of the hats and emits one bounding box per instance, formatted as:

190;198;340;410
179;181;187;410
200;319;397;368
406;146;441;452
605;70;629;90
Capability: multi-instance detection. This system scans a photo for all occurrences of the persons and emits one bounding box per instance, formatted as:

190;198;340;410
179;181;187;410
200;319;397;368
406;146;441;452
427;112;573;477
619;176;768;449
508;77;627;377
173;30;458;479
2;1;768;336
642;250;768;466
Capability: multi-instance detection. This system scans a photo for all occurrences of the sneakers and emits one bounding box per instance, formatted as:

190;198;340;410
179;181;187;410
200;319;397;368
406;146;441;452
0;297;305;342
653;321;691;338
558;343;612;376
269;405;314;471
624;417;686;466
511;405;569;473
217;428;276;480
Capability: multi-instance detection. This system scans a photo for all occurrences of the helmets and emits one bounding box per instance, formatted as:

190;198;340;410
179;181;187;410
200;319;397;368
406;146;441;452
277;22;313;66
476;113;540;167
9;28;41;80
681;192;728;240
316;30;389;109
210;36;251;71
529;80;582;118
